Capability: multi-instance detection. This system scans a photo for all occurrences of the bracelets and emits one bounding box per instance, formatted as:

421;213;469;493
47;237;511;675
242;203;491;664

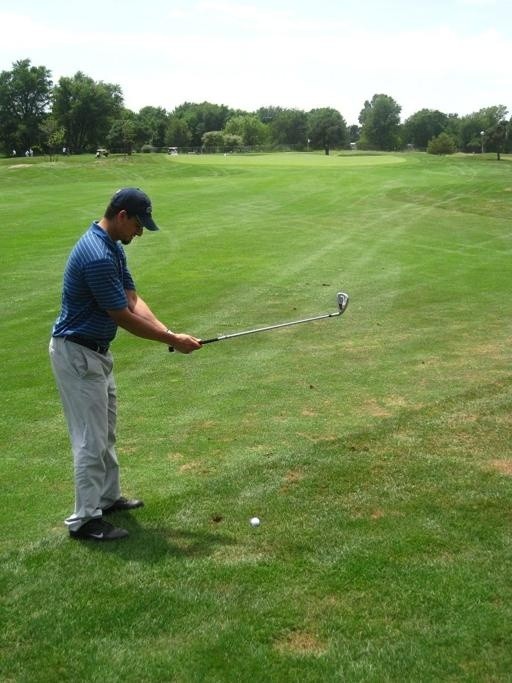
166;328;171;332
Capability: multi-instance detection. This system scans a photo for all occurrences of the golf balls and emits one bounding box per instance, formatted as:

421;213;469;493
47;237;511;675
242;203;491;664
251;517;261;527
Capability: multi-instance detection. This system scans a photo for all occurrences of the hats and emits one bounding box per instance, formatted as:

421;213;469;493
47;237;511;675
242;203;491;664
112;188;159;231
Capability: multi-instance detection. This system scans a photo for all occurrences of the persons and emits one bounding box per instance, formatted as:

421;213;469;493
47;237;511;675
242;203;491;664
47;188;202;541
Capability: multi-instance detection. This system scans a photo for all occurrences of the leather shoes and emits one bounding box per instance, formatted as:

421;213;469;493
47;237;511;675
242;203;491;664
102;496;144;513
68;518;128;541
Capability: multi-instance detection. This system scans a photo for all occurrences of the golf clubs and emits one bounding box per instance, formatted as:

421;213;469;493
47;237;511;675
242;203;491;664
169;291;349;355
